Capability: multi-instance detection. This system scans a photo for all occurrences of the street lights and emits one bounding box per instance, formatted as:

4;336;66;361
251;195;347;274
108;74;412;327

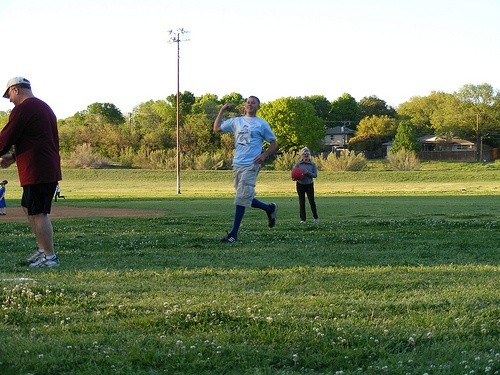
166;27;190;194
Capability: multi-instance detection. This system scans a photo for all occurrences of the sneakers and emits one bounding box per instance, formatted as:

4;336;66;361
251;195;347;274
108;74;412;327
267;203;277;227
221;230;237;243
27;251;60;268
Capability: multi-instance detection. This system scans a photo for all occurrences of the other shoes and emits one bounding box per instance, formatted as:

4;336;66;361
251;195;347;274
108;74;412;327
314;219;320;222
0;213;7;215
300;220;306;223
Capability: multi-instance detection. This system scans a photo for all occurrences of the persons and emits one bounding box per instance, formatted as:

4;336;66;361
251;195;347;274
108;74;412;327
214;95;278;243
0;180;8;215
54;183;65;202
0;77;62;268
292;147;319;223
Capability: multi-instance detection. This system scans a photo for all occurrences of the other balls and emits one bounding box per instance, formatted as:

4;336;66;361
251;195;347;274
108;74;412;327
291;167;305;181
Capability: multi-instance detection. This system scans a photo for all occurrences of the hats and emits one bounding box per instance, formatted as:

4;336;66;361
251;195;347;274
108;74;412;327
3;77;31;98
301;147;310;154
